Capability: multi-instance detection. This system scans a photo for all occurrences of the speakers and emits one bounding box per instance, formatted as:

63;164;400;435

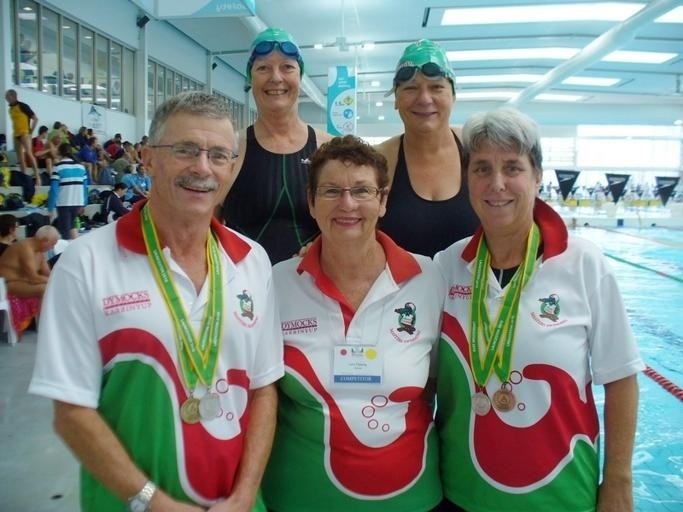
245;86;251;92
137;16;150;27
213;63;217;69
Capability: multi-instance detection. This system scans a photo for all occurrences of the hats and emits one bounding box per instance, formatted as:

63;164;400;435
244;27;305;84
392;38;456;96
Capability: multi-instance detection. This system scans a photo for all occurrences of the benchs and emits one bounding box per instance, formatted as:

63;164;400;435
0;147;148;255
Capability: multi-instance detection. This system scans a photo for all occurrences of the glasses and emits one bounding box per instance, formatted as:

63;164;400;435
393;61;451;84
312;185;382;202
248;40;302;66
149;141;239;166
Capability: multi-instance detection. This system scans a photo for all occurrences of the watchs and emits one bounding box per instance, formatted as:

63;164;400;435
125;480;157;512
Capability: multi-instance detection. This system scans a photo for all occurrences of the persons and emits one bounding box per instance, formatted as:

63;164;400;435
27;91;284;512
213;27;334;266
258;134;444;512
370;38;480;260
0;90;151;298
291;107;647;512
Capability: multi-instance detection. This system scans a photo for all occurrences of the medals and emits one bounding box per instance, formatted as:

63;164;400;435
472;387;492;417
492;383;516;412
198;393;221;420
180;393;200;424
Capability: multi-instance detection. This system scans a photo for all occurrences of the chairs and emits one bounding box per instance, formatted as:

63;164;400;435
0;278;39;347
